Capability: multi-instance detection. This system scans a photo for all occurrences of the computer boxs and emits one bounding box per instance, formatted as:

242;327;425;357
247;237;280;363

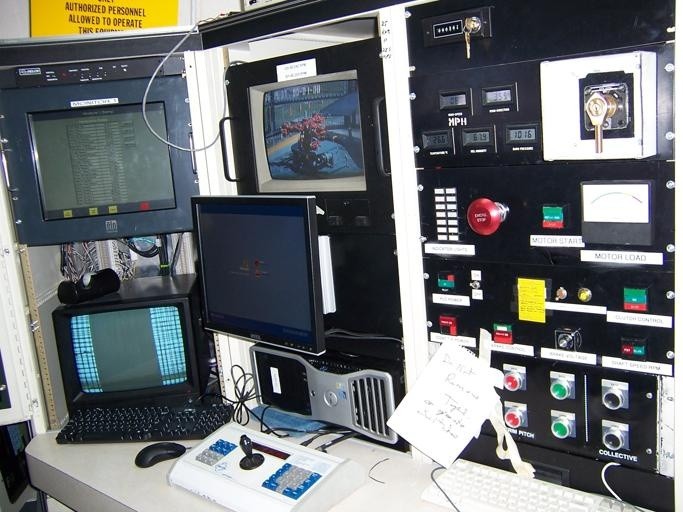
249;342;405;446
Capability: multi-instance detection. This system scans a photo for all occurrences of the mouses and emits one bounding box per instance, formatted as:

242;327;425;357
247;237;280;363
135;442;186;468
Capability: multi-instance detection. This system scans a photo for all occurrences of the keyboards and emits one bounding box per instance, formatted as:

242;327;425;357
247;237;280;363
56;403;234;444
421;458;655;512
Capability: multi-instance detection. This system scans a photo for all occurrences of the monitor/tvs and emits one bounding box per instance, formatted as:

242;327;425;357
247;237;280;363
190;195;327;357
27;102;176;221
52;273;211;420
245;68;367;192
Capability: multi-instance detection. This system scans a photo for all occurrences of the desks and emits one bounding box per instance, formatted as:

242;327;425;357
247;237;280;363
24;400;664;511
